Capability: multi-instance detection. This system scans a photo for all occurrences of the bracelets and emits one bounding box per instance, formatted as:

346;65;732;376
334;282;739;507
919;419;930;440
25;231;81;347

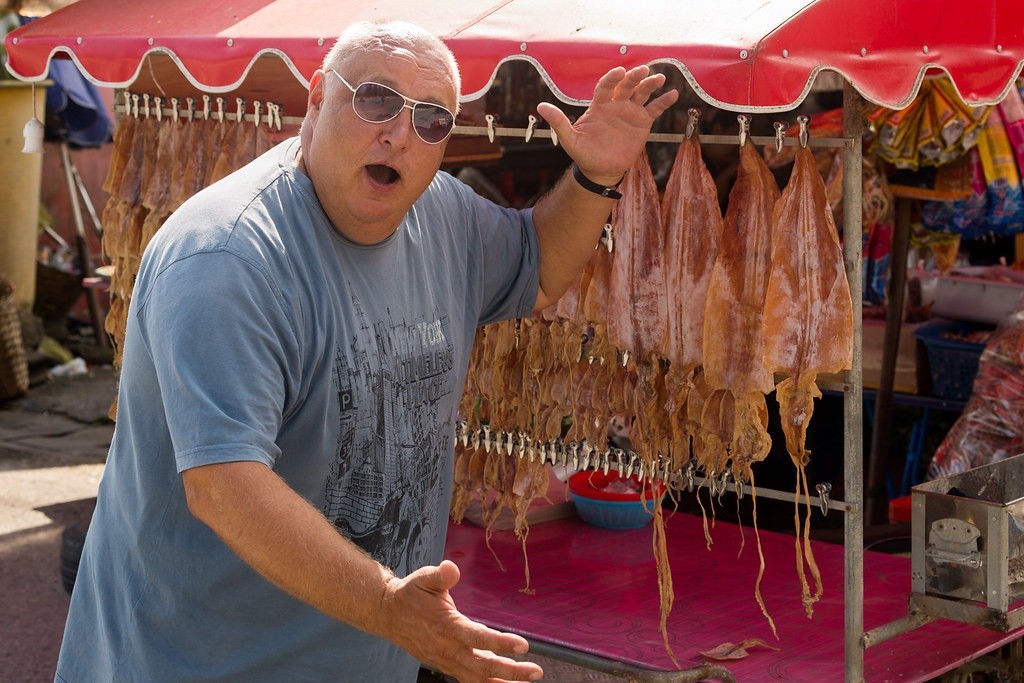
572;160;627;200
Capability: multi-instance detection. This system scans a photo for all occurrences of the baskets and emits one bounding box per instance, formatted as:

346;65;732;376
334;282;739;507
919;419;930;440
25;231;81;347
912;317;995;404
566;489;667;529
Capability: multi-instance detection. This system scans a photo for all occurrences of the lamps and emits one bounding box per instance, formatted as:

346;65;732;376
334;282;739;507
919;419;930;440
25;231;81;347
21;116;47;153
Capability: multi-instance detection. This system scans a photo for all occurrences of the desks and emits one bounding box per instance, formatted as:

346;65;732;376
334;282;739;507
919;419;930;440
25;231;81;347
460;481;1023;683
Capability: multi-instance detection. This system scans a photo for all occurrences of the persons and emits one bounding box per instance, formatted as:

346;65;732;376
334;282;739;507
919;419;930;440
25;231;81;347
53;17;679;683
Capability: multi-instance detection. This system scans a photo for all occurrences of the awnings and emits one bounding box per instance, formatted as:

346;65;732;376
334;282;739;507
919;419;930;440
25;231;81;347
0;1;1021;116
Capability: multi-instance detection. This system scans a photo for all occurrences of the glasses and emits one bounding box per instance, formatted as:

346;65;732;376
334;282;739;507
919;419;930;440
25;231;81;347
326;66;457;144
